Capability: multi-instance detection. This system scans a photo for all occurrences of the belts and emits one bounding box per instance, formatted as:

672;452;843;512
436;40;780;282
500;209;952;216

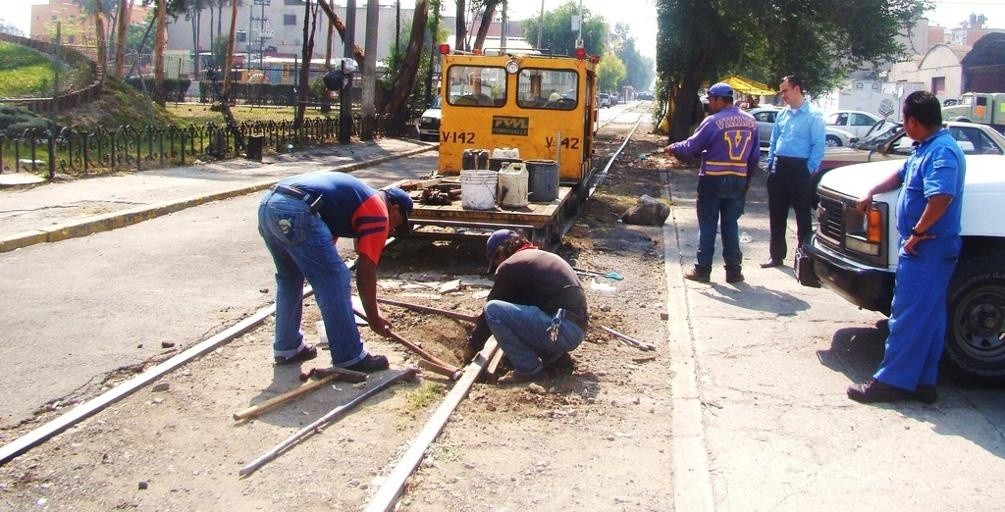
270;183;324;213
552;309;589;332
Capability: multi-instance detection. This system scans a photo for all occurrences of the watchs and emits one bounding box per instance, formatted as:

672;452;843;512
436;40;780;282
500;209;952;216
910;226;924;238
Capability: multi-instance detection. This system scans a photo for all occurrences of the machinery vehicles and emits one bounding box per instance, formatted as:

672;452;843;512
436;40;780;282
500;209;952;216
381;47;600;245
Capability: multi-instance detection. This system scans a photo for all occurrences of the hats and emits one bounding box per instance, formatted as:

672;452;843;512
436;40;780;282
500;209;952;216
708;83;734;98
486;228;511;274
385;186;413;236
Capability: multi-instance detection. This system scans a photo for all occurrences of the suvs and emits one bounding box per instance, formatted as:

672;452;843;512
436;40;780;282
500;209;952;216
795;153;1005;391
600;92;611;108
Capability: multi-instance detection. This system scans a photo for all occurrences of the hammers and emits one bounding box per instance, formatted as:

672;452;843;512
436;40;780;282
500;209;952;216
232;366;367;422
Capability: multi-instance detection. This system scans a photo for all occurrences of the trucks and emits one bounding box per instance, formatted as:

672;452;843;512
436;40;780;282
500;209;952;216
942;92;1005;140
636;92;653;101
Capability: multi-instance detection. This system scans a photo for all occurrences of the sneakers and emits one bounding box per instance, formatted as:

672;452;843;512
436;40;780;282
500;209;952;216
274;344;317;363
333;353;389;372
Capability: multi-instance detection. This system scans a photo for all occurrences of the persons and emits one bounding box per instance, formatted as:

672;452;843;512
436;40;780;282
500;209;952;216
760;74;826;269
258;170;413;373
463;229;588;385
548;88;560;102
662;83;761;283
846;91;966;403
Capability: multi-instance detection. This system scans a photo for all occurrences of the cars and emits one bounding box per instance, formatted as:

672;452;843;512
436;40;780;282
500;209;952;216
825;110;901;147
811;121;1004;210
743;107;860;148
419;94;441;141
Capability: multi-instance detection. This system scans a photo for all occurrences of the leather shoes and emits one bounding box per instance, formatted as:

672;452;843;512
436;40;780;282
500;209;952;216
544;353;575;367
760;257;783;267
685;268;709;281
848;378;913;403
726;273;745;283
499;369;541;383
904;382;936;402
